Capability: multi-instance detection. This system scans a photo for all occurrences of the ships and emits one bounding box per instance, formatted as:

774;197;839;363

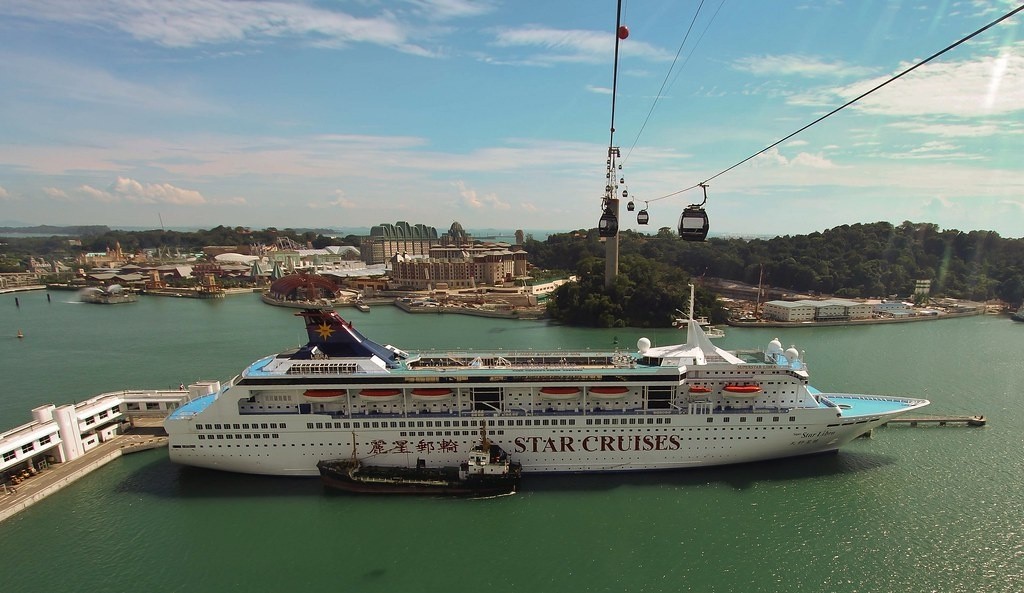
163;285;932;481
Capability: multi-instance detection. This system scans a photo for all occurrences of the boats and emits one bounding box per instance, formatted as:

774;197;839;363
315;418;522;497
720;385;763;398
355;300;370;313
588;387;631;399
409;388;453;401
17;329;24;339
359;389;403;403
687;386;714;396
320;298;335;314
82;286;138;305
703;325;725;339
671;308;710;330
538;387;582;400
302;390;346;403
